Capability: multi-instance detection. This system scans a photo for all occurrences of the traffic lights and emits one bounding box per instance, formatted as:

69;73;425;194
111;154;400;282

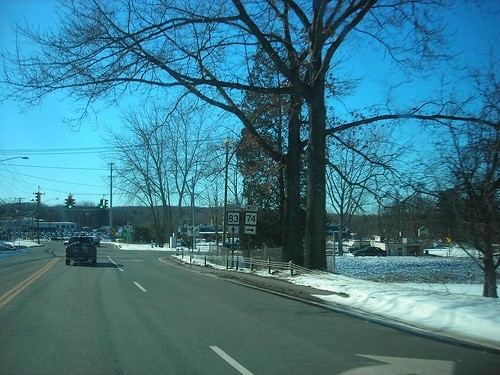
35;192;38;202
99;199;103;209
71;198;76;208
105;199;109;208
64;198;70;208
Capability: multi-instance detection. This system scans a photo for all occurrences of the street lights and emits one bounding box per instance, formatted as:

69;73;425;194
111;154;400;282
108;162;115;235
222;140;230;249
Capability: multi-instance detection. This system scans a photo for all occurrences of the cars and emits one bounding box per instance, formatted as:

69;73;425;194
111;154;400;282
64;232;101;248
64;236;97;266
352;246;387;257
333;244;351;254
0;243;17;251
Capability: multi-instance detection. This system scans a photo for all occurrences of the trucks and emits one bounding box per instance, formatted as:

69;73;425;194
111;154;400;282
347;240;371;253
